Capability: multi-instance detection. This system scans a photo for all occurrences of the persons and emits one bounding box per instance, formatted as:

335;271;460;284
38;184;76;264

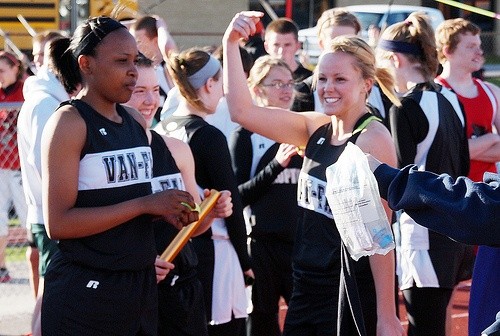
158;48;254;336
222;11;405;336
0;9;500;303
41;14;199;336
364;153;500;336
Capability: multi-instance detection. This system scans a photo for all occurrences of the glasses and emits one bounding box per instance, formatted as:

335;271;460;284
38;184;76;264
259;80;295;90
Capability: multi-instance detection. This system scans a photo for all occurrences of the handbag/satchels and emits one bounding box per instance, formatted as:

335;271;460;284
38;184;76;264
324;140;396;261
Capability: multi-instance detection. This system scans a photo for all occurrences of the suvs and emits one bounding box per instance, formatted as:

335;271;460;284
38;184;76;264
293;4;446;70
